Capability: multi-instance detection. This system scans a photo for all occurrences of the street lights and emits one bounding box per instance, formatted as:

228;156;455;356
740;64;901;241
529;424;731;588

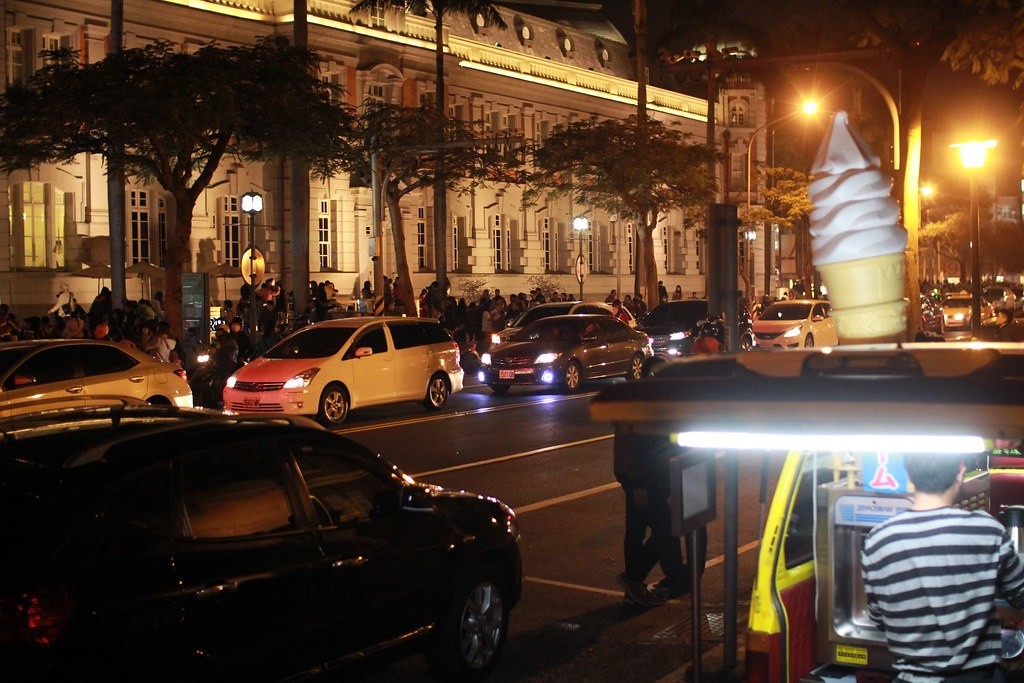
948;130;998;328
746;101;818;316
241;188;263;350
573;213;588;301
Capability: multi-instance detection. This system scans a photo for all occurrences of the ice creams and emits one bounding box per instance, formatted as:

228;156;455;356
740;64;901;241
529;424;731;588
805;110;911;344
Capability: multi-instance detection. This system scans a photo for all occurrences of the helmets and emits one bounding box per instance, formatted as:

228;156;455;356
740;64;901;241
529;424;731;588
215;324;230;334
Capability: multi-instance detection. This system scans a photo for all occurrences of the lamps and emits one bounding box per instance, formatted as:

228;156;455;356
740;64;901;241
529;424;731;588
668;429;992;456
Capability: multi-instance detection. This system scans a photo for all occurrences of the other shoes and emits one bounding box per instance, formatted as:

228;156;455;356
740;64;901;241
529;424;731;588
617;574;666;605
653;576;690;598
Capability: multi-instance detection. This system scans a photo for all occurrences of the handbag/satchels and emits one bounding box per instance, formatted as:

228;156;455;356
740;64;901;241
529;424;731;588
62;303;72;314
170;350;179;363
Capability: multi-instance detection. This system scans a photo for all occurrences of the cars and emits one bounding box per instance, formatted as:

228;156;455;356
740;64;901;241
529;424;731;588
477;313;654;396
488;301;614;353
606;303;637;327
0;337;194;421
223;314;465;425
982;286;1017;308
633;298;753;361
752;299;839;350
939;290;995;331
0;404;524;683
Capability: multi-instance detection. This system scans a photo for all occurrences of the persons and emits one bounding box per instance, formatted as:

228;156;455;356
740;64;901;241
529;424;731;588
653;434;706;596
738;282;822;335
861;453;1024;683
919;279;1014;331
602;281;708;350
0;274;577;413
610;356;674;605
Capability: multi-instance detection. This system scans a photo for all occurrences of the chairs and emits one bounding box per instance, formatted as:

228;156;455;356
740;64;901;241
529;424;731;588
189;476;294;537
